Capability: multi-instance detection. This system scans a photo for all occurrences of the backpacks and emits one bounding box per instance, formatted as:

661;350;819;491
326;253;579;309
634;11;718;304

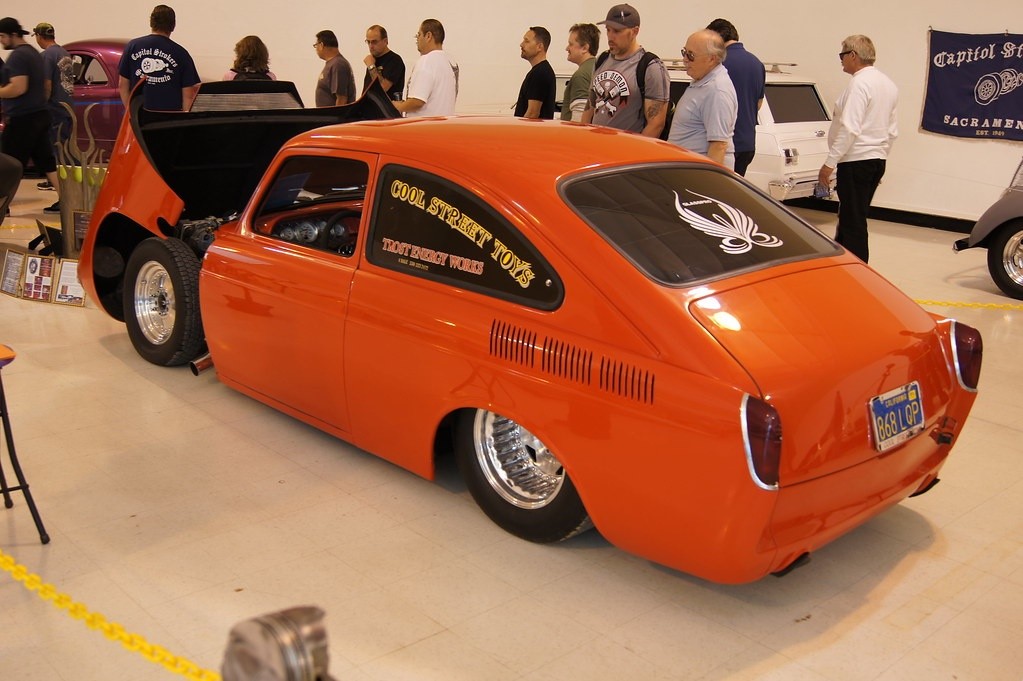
595;50;676;141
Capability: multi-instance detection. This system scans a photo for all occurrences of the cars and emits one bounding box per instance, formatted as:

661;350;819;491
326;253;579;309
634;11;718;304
512;59;838;205
954;162;1023;300
76;80;982;585
60;38;132;158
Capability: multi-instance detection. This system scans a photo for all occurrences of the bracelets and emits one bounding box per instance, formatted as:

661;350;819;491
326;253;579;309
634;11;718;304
367;64;376;70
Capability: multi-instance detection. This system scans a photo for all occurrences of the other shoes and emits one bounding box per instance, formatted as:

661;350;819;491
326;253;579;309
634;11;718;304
43;200;60;214
4;208;10;216
36;181;56;191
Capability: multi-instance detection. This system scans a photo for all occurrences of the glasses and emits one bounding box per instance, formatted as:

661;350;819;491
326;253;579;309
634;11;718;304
313;41;324;48
365;39;381;44
681;49;704;62
839;50;857;60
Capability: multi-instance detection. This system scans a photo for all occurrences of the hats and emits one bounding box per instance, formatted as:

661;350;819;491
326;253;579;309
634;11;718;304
0;17;29;35
596;3;640;30
31;22;54;37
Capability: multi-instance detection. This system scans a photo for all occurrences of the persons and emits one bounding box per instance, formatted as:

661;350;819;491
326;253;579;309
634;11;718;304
362;25;405;101
513;26;556;119
0;17;74;217
706;18;766;178
116;4;202;113
666;28;738;171
561;23;601;123
392;19;459;117
817;35;899;266
222;35;277;81
313;30;356;107
581;3;671;138
